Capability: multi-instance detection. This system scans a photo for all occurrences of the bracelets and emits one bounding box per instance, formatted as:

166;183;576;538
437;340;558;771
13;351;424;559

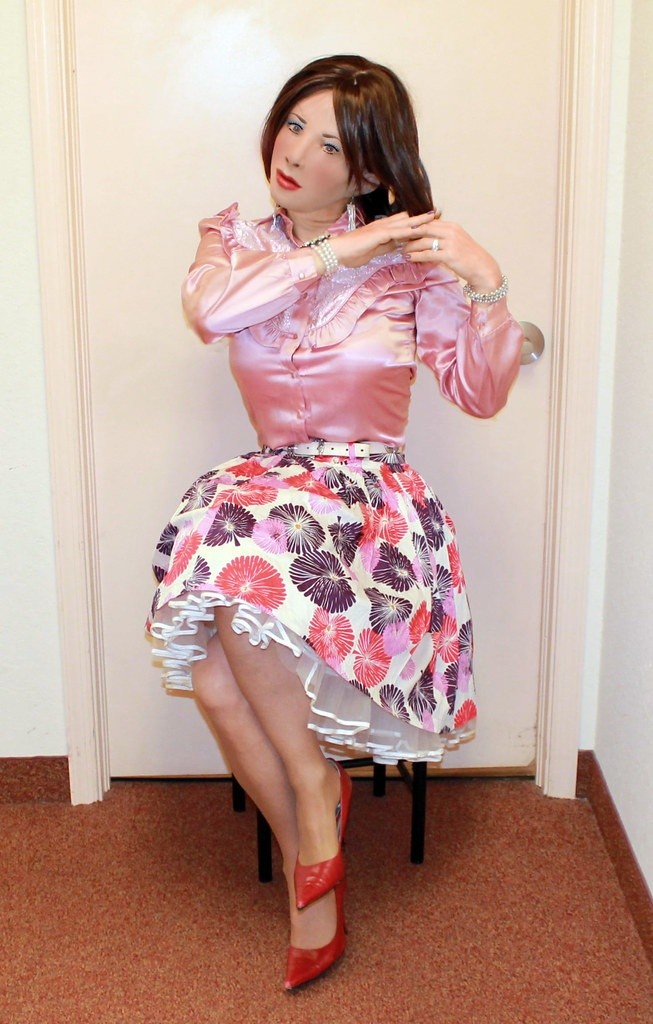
462;276;508;304
301;233;340;279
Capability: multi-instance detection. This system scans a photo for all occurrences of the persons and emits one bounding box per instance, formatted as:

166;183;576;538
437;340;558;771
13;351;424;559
141;50;523;990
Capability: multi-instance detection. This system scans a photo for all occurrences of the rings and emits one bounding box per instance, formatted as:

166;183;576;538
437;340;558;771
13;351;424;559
432;236;439;251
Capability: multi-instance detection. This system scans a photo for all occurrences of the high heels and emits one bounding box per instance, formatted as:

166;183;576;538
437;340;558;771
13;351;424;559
282;866;348;991
294;757;353;910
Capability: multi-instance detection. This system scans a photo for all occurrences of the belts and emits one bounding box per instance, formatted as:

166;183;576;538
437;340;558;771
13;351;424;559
265;440;406;458
